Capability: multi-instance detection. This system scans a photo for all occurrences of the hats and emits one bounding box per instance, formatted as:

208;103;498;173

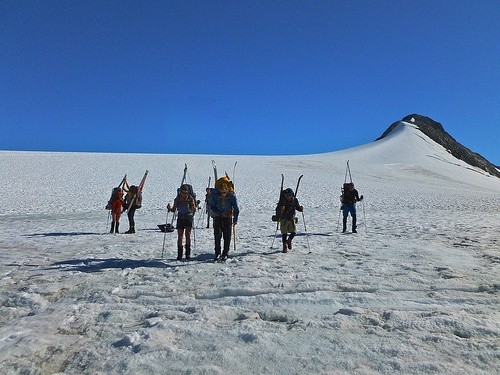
181;184;189;192
284;188;293;195
346;183;354;188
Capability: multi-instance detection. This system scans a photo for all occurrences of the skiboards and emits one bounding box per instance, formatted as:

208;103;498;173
195;176;212;229
344;159;353;184
169;162;202;229
276;173;304;230
123;169;149;214
117;173;128;194
212;160;238;189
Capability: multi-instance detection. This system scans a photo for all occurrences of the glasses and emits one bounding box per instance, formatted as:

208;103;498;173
285;194;292;196
182;192;187;194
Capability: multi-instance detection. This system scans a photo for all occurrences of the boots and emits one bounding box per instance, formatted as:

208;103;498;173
115;222;119;233
214;246;221;261
286;238;292;249
222;246;229;261
185;244;190;260
132;220;135;233
125;220;133;233
177;245;183;260
282;238;287;253
110;222;115;233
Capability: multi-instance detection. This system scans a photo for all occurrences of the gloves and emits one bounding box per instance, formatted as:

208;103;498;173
277;205;283;212
360;195;363;199
299;206;303;212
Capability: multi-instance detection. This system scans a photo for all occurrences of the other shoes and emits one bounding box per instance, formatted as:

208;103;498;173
352;226;357;233
342;227;346;233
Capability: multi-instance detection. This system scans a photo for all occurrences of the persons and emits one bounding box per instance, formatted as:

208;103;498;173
210;177;239;261
339;183;363;233
276;188;303;253
167;184;196;261
110;187;124;234
205;188;214;228
125;185;137;233
